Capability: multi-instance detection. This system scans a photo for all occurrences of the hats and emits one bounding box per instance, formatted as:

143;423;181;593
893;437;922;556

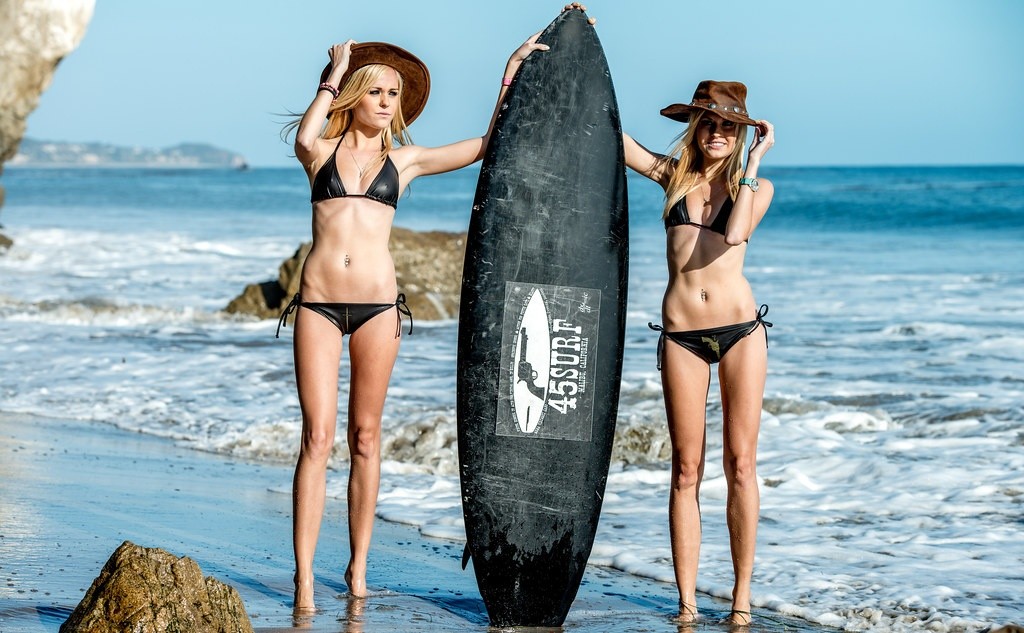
319;42;431;135
660;80;757;126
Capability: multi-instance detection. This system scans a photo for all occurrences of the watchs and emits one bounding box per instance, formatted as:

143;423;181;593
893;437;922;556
738;177;759;193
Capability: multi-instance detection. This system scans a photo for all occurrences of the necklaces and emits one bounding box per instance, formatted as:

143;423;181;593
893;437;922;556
695;171;729;204
338;132;386;177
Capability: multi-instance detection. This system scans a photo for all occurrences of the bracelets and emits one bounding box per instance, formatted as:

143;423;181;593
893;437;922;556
318;81;340;99
501;77;513;86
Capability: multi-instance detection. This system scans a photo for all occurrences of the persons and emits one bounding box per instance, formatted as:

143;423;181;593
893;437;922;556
275;25;551;612
560;1;777;626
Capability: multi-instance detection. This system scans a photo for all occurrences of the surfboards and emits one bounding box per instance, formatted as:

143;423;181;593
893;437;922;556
452;3;631;629
